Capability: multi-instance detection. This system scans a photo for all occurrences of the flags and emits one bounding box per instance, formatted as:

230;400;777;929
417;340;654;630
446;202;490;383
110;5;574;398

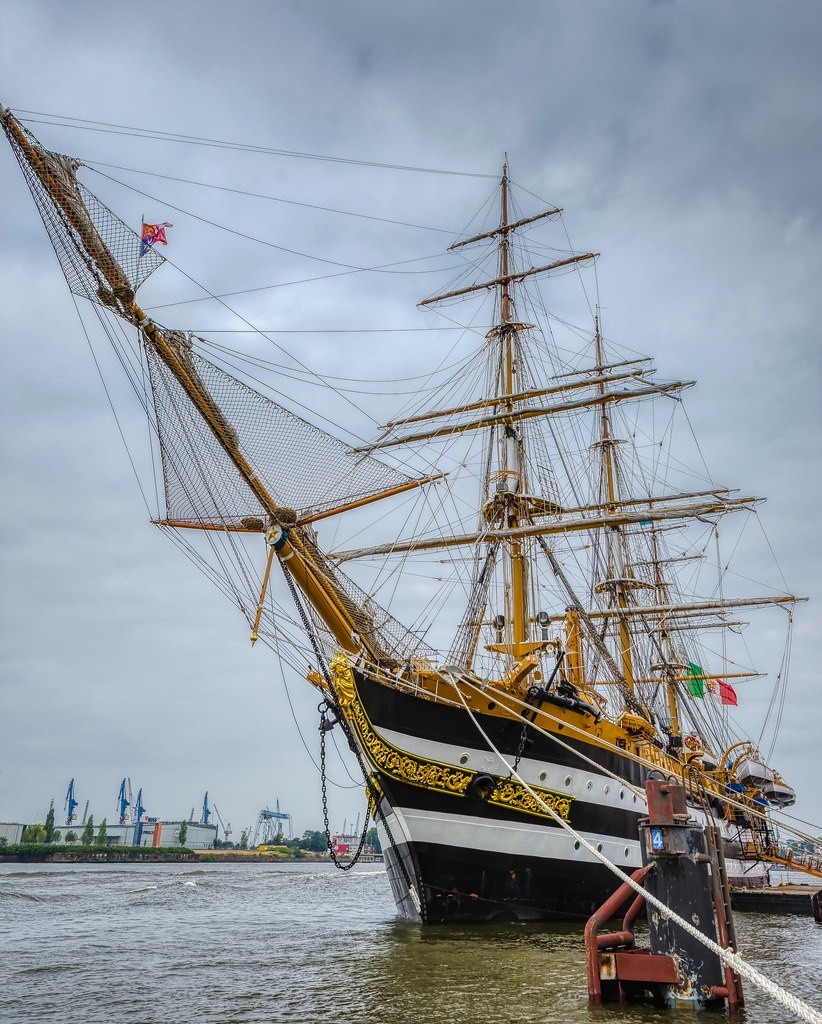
687;661;739;707
143;222;173;245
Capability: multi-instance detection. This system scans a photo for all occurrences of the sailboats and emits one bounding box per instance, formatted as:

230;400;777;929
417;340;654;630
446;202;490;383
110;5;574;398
0;101;822;923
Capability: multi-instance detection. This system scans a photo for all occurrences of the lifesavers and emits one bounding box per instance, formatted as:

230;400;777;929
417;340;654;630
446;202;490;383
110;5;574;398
689;740;700;751
685;736;697;748
